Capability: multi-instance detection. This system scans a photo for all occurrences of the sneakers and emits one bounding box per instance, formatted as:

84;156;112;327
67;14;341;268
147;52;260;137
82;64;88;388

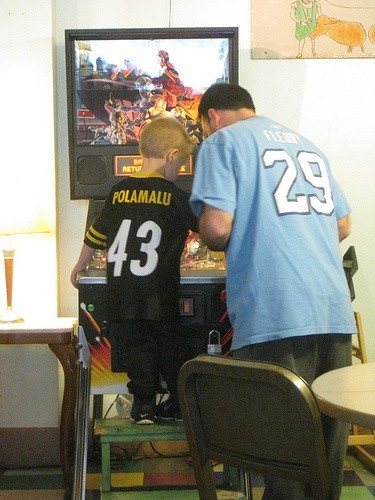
130;405;154;424
154;399;182;420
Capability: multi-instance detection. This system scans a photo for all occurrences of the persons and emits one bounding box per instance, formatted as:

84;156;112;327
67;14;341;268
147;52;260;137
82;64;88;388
188;83;359;500
70;115;200;424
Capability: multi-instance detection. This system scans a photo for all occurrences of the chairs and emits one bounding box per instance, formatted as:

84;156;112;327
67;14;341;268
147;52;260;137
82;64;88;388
177;354;332;500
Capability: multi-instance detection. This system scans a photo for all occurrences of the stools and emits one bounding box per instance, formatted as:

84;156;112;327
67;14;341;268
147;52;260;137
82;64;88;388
93;418;240;492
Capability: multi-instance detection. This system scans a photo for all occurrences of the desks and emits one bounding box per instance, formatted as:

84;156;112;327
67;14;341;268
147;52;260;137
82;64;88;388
311;362;375;430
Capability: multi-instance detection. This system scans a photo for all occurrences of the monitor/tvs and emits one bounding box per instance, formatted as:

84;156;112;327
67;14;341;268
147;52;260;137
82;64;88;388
90;228;227;271
